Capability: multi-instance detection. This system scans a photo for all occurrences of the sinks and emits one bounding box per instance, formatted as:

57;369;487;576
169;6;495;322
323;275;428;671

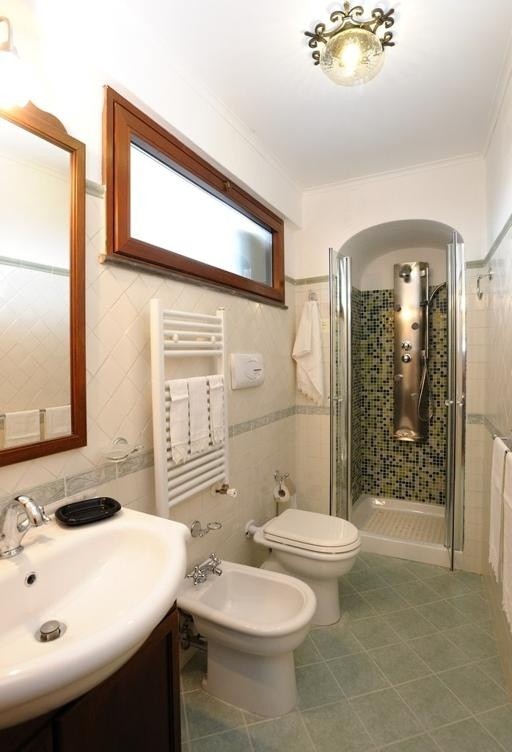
178;560;317;656
0;507;192;728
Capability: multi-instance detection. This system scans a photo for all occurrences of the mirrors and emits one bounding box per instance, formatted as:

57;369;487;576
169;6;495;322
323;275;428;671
100;83;287;311
0;99;89;469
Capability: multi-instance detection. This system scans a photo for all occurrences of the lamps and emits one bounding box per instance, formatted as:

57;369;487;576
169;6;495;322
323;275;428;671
0;11;32;112
304;2;401;90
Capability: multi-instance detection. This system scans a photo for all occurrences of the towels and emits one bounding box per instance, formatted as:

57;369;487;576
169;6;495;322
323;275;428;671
499;447;512;629
291;300;326;408
168;379;189;466
189;376;210;455
488;433;510;585
2;408;42;449
208;374;226;445
42;404;72;443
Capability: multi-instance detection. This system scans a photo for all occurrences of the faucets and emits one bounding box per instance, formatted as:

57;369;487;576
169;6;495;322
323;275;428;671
188;552;223;586
0;494;52;559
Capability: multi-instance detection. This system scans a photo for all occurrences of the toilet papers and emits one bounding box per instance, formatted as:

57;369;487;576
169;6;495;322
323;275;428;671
274;480;297;503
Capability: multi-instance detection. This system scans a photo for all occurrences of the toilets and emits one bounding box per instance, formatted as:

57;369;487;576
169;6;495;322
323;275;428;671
253;508;362;626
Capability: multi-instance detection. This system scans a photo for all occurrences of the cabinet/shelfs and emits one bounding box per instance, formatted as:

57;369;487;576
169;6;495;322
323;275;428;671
0;604;185;752
